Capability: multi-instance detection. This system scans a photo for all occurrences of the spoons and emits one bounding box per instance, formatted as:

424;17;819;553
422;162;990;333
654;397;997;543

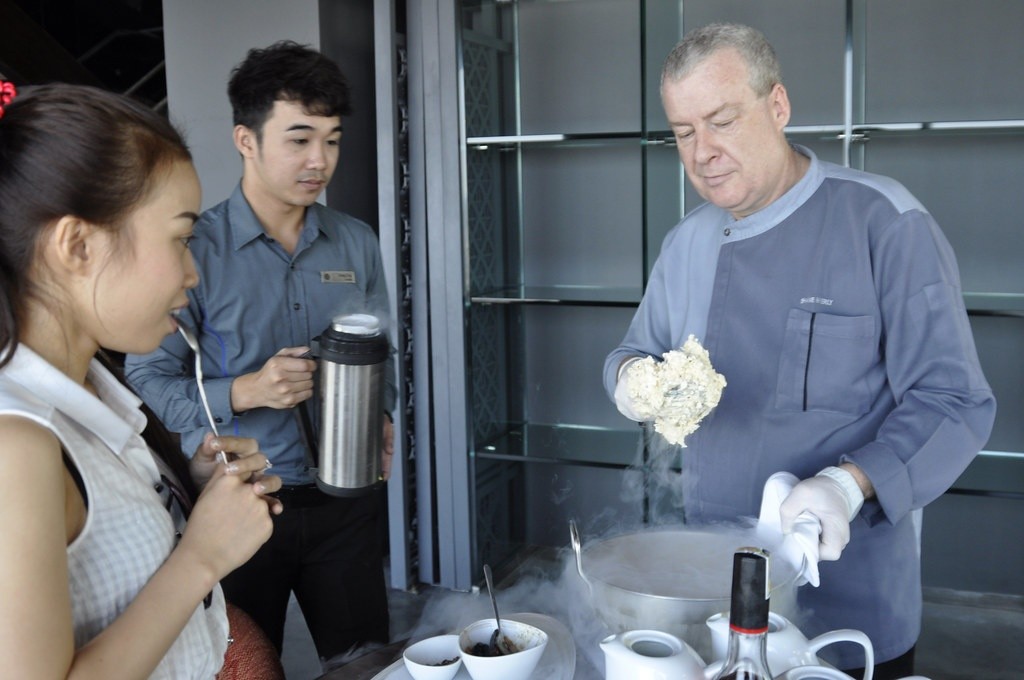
483;564;511;654
170;311;233;465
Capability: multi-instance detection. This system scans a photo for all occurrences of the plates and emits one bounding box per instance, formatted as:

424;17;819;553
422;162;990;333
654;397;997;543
370;613;576;680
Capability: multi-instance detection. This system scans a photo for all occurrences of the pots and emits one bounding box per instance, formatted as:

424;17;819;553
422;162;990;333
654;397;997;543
569;519;808;664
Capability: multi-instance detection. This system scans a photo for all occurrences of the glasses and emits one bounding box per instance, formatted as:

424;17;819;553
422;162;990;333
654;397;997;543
159;474;213;609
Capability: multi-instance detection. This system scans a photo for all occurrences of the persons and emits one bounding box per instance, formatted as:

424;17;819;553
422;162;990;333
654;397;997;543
123;39;397;680
603;21;997;680
0;80;284;680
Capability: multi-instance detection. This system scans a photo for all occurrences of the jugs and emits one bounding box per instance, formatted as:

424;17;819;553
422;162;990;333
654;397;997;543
600;629;725;680
706;612;874;680
774;665;932;680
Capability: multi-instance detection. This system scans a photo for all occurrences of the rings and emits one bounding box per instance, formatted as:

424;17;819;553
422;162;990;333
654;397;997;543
256;460;273;473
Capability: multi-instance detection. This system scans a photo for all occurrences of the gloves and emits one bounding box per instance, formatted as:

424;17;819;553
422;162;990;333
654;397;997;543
614;357;654;423
778;467;864;564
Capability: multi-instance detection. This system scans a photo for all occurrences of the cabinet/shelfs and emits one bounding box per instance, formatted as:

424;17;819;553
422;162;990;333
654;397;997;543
453;0;1024;680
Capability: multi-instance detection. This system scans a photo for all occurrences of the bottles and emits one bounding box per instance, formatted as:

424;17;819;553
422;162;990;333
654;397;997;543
713;546;773;680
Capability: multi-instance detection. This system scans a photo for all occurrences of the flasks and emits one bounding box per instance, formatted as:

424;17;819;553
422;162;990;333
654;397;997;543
296;312;399;495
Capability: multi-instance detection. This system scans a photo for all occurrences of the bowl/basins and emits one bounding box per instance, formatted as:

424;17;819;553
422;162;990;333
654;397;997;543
458;618;549;680
403;635;462;680
